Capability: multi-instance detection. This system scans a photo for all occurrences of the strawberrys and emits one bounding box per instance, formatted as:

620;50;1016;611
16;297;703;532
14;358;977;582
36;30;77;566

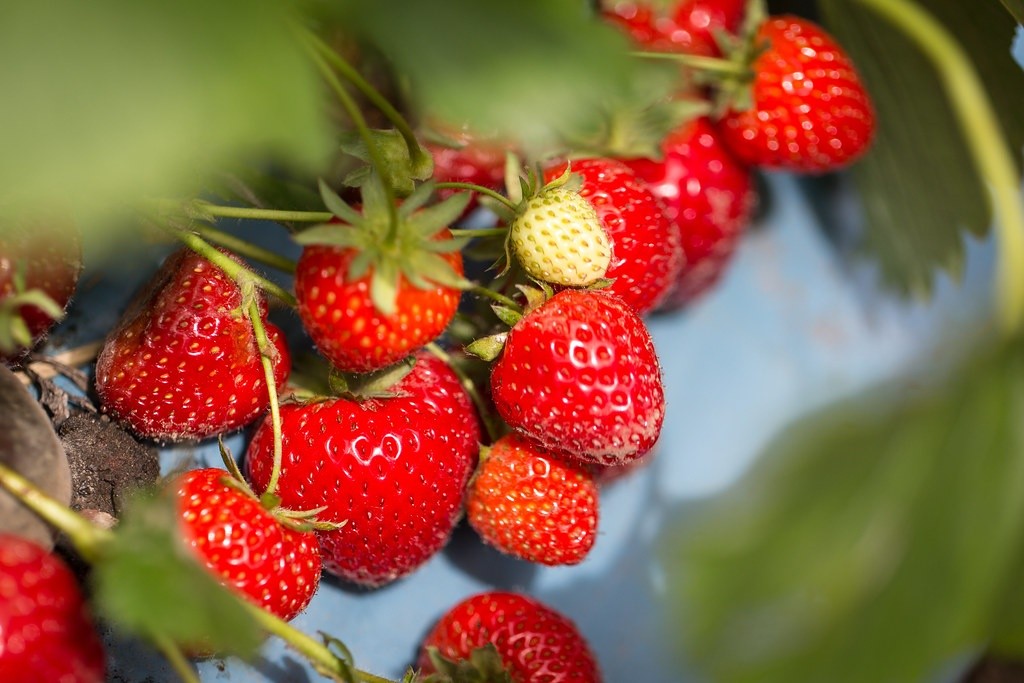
1;1;874;683
